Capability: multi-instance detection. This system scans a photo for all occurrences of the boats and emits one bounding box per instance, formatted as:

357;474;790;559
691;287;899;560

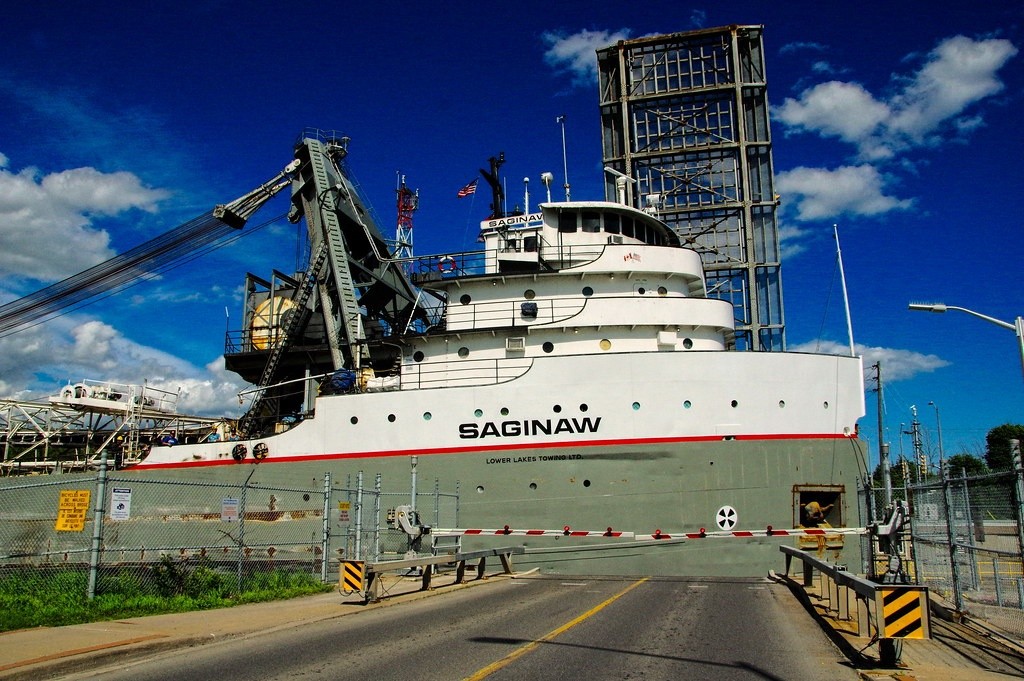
0;130;873;576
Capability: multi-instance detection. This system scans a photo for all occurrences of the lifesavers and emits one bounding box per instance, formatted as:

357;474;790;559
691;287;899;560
438;256;455;273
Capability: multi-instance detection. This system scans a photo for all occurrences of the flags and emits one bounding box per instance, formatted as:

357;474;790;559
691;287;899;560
456;180;477;198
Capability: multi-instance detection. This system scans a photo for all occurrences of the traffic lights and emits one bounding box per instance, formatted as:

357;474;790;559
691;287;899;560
901;463;907;480
921;456;927;474
886;427;895;468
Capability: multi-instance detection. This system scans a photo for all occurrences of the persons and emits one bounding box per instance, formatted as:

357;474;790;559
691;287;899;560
223;427;239;441
208;425;221;442
163;431;178;448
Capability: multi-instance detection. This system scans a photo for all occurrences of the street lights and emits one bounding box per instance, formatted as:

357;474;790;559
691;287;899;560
928;400;946;481
899;423;904;463
866;436;872;474
908;304;1024;374
